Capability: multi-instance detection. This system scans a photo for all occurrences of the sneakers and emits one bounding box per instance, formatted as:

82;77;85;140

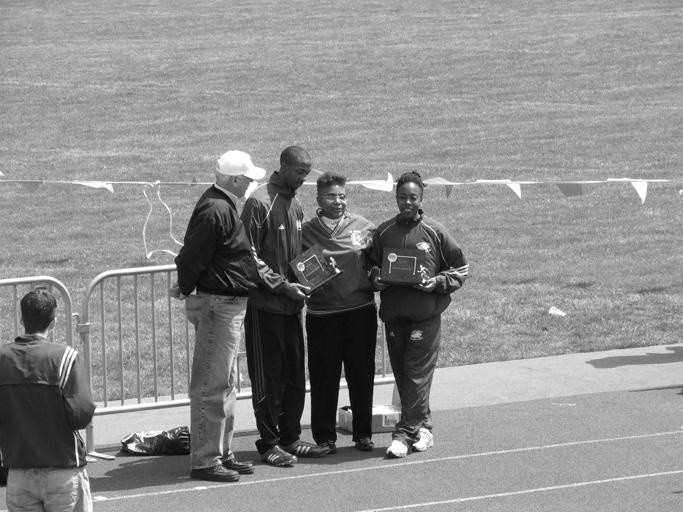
223;458;255;474
411;428;434;451
190;464;240;482
386;440;407;457
281;440;330;458
356;437;373;451
260;445;297;466
319;441;336;452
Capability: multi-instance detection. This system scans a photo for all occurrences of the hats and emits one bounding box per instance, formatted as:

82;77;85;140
216;150;266;179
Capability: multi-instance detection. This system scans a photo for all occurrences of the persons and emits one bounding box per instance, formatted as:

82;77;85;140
365;172;469;457
168;150;266;483
301;171;378;454
240;147;330;466
0;289;96;512
